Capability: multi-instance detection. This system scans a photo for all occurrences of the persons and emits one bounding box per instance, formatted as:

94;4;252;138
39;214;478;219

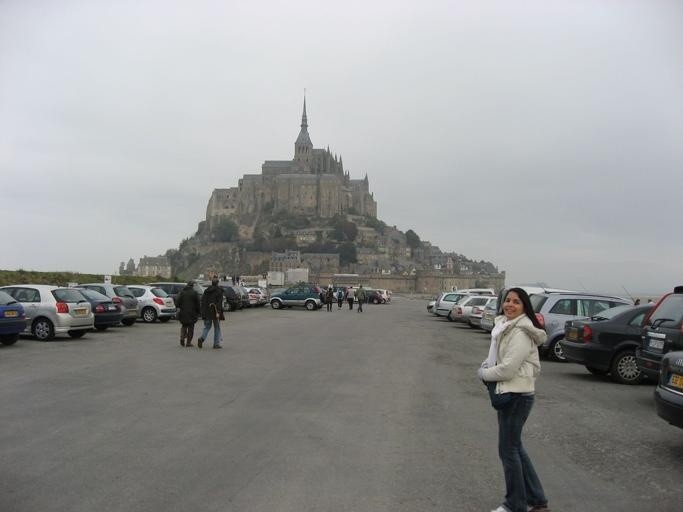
175;281;201;347
325;288;333;312
336;288;344;309
354;285;366;312
477;287;548;512
197;278;225;349
345;286;355;309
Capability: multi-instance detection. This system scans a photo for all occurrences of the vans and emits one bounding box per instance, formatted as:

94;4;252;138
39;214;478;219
0;284;94;342
72;282;138;326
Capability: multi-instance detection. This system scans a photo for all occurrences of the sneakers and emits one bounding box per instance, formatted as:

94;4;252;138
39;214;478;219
198;338;223;348
489;505;513;512
527;504;549;512
180;338;194;349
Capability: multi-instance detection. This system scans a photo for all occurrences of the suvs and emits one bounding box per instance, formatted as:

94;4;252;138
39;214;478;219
528;291;635;363
634;285;683;386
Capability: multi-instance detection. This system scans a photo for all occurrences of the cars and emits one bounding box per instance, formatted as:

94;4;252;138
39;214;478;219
654;350;683;429
559;303;657;385
71;287;123;331
122;277;392;323
426;287;576;334
0;290;27;346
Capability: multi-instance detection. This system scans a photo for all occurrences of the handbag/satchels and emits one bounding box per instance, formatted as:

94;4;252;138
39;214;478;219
481;368;522;409
